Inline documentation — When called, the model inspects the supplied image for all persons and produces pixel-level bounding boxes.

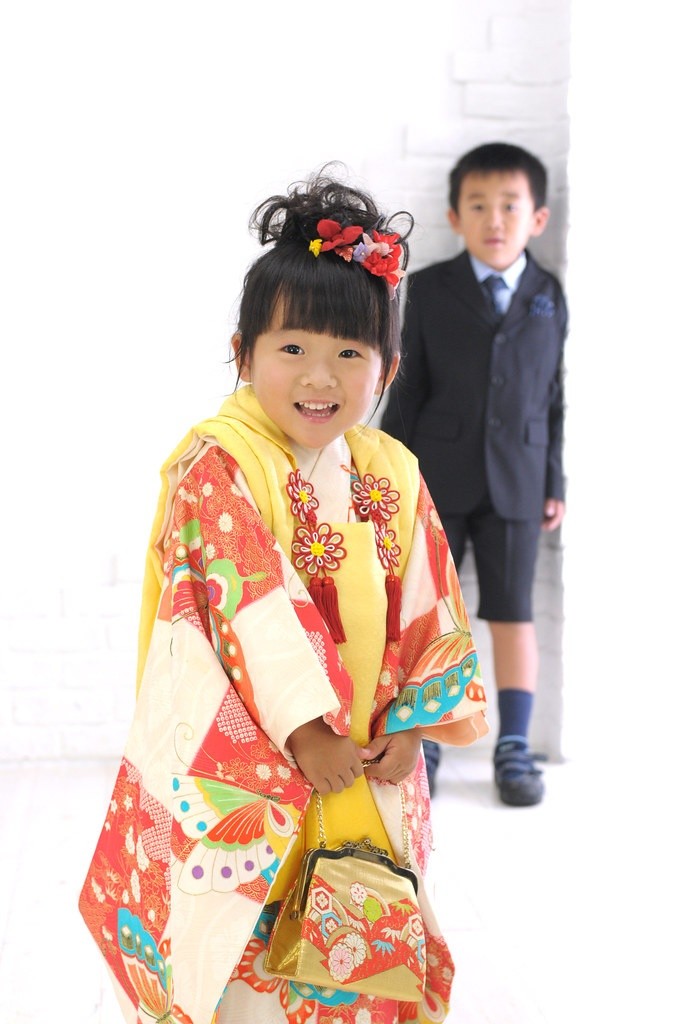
[77,182,487,1024]
[378,140,567,808]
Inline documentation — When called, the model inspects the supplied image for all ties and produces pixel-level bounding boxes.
[483,275,508,319]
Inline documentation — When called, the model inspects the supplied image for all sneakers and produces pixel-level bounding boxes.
[492,741,543,804]
[424,740,441,796]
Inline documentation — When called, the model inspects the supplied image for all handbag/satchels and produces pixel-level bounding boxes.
[264,765,425,1002]
